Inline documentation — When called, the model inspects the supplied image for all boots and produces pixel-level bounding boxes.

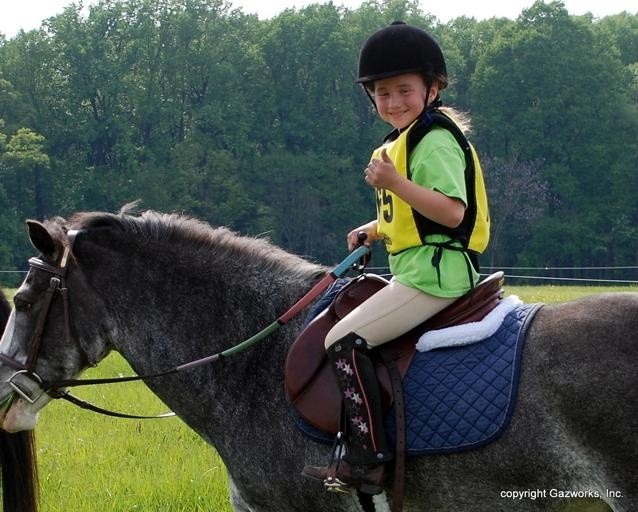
[301,333,390,495]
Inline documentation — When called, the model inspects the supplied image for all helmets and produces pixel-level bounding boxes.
[356,21,448,90]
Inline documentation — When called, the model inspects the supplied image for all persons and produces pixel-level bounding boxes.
[301,21,492,496]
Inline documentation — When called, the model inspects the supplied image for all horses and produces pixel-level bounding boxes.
[0,198,637,511]
